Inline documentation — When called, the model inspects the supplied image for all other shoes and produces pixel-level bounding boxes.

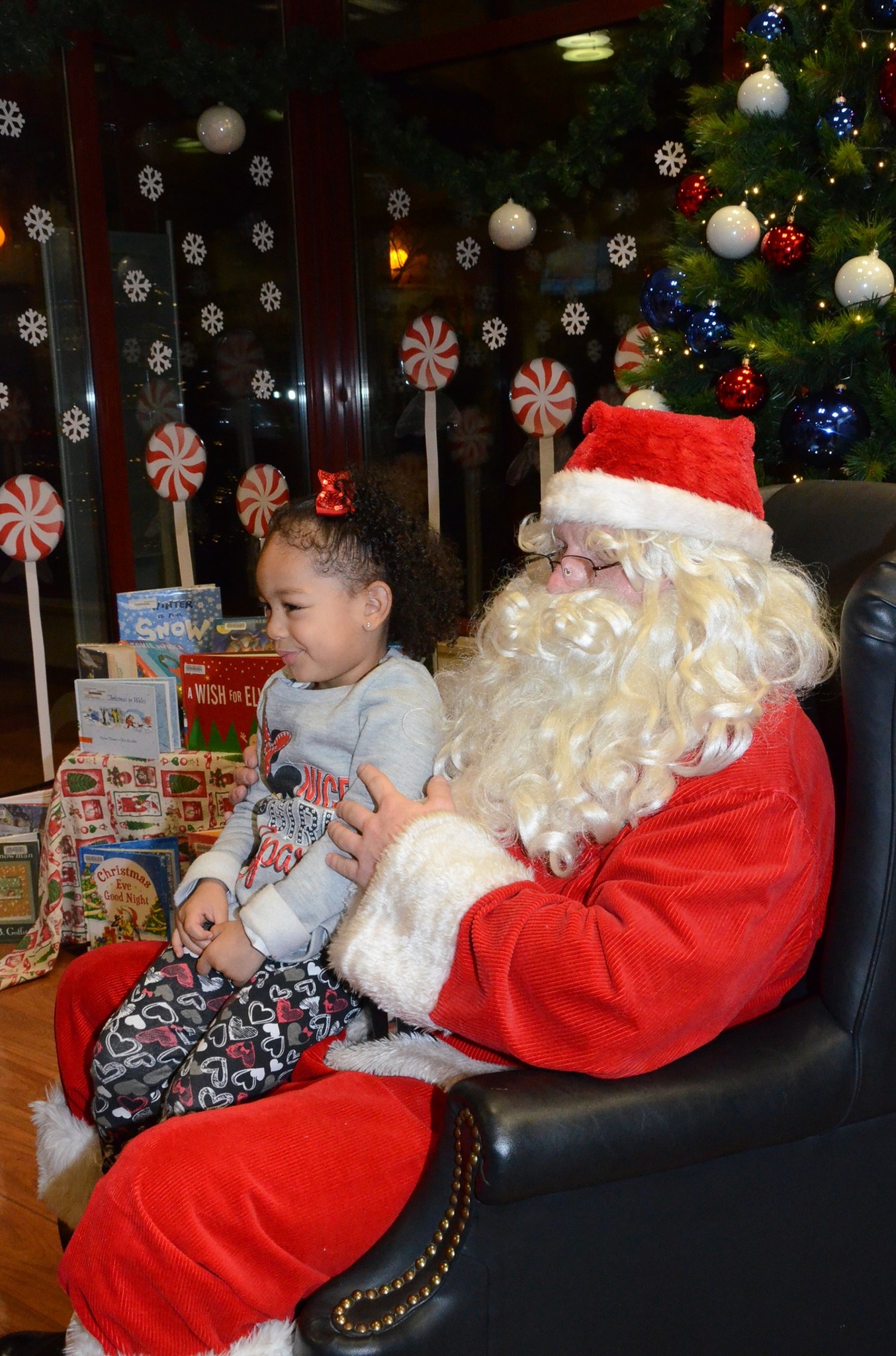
[0,1330,67,1356]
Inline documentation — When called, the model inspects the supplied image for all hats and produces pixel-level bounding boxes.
[538,400,773,563]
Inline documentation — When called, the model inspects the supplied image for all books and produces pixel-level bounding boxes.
[116,582,223,654]
[74,675,181,760]
[211,616,277,654]
[0,831,41,943]
[78,836,181,953]
[187,824,227,866]
[0,788,55,837]
[179,649,288,754]
[76,641,138,679]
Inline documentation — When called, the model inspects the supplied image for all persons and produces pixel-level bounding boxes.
[24,395,844,1356]
[86,465,466,1178]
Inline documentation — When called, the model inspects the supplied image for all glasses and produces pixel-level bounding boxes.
[517,545,621,590]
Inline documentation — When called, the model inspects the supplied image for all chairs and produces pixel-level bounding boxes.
[291,478,896,1353]
